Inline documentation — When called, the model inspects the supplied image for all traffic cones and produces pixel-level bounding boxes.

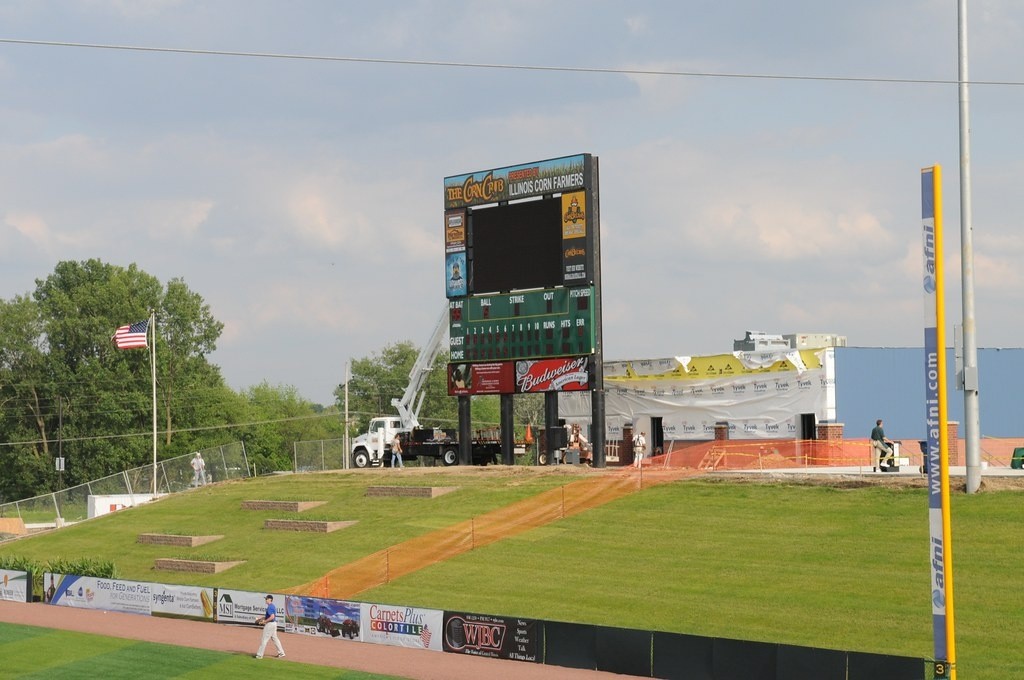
[525,424,533,442]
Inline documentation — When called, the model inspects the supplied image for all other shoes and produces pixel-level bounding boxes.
[400,465,405,468]
[880,462,890,467]
[875,469,882,473]
[255,655,263,659]
[275,654,286,658]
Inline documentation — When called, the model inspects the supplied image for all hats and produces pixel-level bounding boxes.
[264,595,273,599]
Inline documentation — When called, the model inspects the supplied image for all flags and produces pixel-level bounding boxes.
[675,355,691,373]
[787,351,807,375]
[115,318,150,348]
[526,424,531,441]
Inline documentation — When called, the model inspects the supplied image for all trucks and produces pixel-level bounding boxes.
[351,300,533,468]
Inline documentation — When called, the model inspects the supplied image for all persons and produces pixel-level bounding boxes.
[871,420,893,471]
[391,434,405,467]
[632,432,645,467]
[654,446,662,455]
[191,453,205,488]
[47,574,55,601]
[254,595,285,659]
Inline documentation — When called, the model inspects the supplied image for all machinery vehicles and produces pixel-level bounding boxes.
[539,423,619,466]
